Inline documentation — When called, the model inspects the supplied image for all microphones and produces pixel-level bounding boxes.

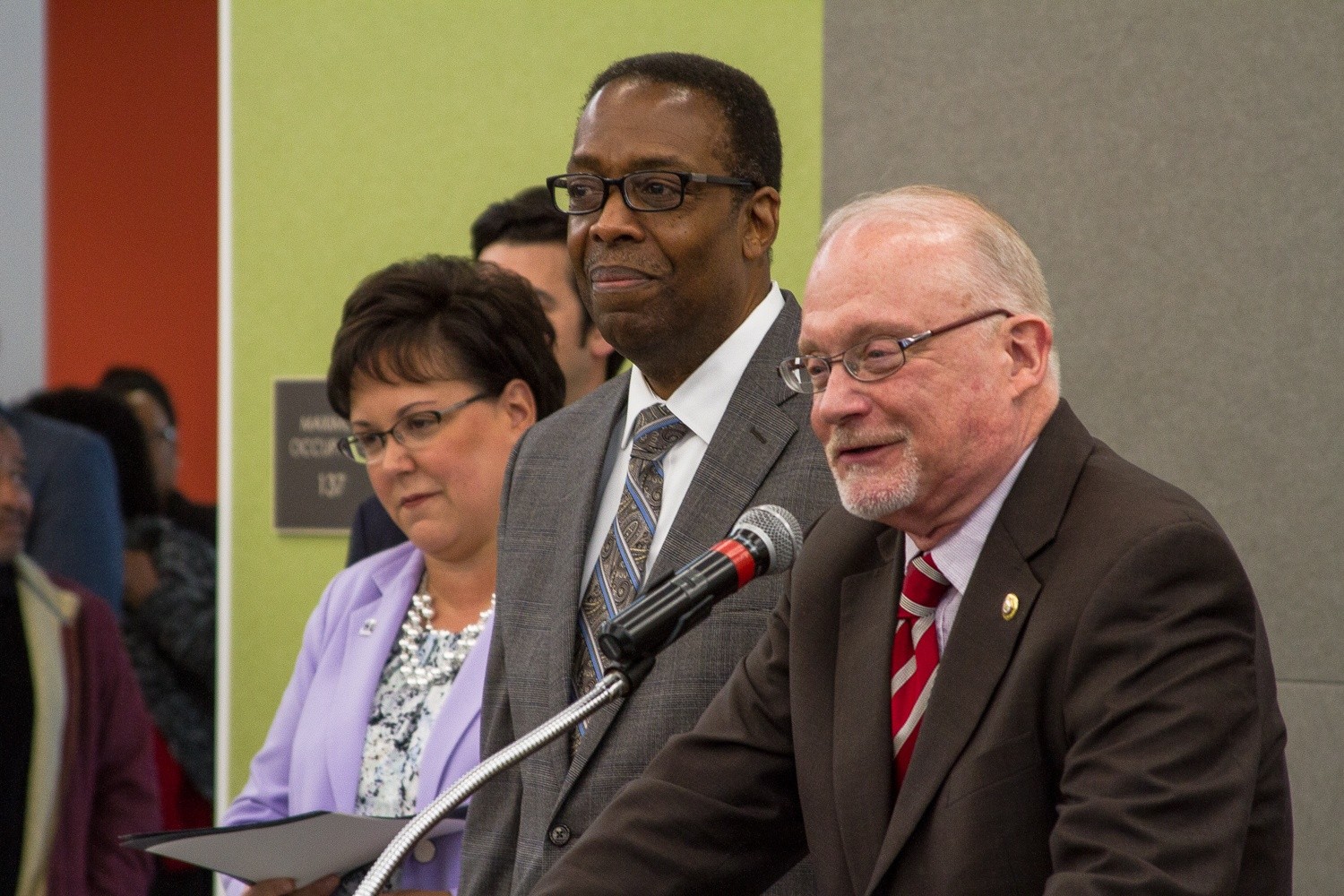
[595,502,804,666]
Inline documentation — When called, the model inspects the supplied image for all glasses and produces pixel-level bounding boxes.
[335,391,503,464]
[546,167,779,213]
[775,308,1015,394]
[145,427,173,447]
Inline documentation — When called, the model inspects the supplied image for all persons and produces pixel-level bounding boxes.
[0,361,215,896]
[525,184,1296,896]
[454,53,847,896]
[345,181,624,573]
[210,254,568,896]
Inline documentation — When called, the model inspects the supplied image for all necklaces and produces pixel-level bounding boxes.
[399,563,499,689]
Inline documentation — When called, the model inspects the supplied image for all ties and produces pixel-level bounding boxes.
[888,551,954,804]
[568,402,690,768]
